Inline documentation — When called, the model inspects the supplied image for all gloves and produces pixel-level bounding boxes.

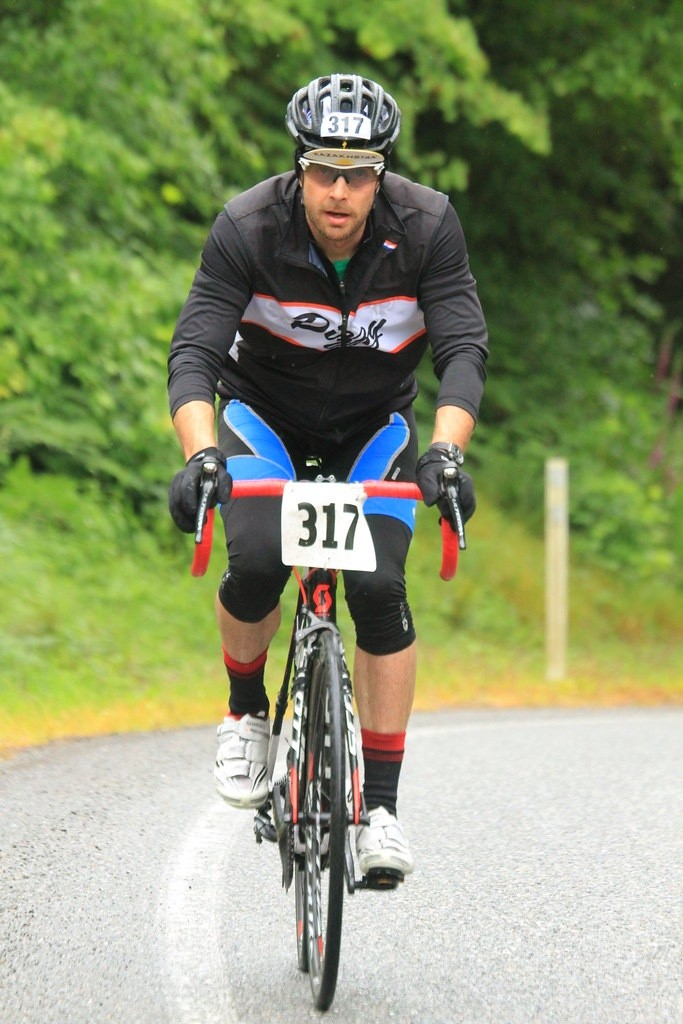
[415,449,476,532]
[169,447,233,533]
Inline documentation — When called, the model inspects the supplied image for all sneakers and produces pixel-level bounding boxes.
[356,805,415,874]
[214,712,269,809]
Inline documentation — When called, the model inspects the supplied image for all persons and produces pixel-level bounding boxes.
[165,74,490,877]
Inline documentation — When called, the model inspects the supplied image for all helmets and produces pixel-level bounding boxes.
[284,73,402,175]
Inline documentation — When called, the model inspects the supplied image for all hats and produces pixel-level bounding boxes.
[301,146,385,168]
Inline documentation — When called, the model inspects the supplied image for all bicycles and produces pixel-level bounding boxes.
[185,444,470,1013]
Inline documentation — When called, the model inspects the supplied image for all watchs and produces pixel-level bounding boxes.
[427,441,466,468]
